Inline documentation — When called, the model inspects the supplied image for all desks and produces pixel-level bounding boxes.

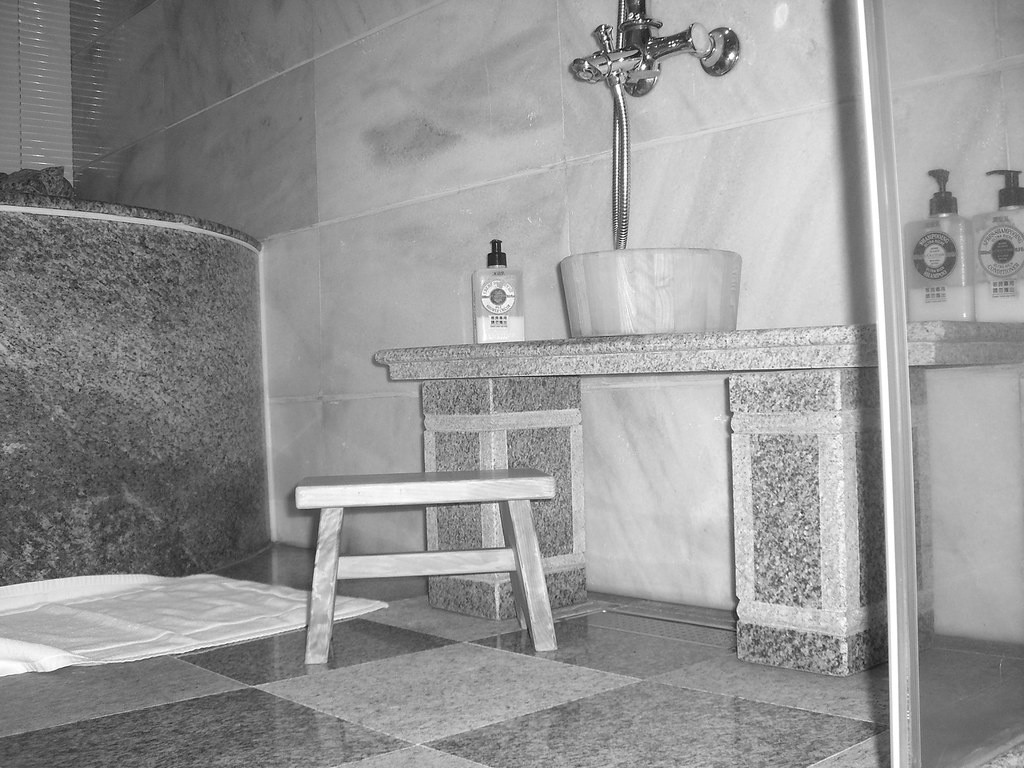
[373,318,1024,678]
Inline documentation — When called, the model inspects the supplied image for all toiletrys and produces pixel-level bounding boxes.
[904,166,972,326]
[469,238,527,344]
[972,168,1024,324]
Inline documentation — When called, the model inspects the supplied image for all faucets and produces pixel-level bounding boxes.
[570,18,662,85]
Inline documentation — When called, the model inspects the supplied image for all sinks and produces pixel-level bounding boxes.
[559,246,740,341]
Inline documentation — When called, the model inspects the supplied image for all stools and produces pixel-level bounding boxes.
[295,470,557,665]
